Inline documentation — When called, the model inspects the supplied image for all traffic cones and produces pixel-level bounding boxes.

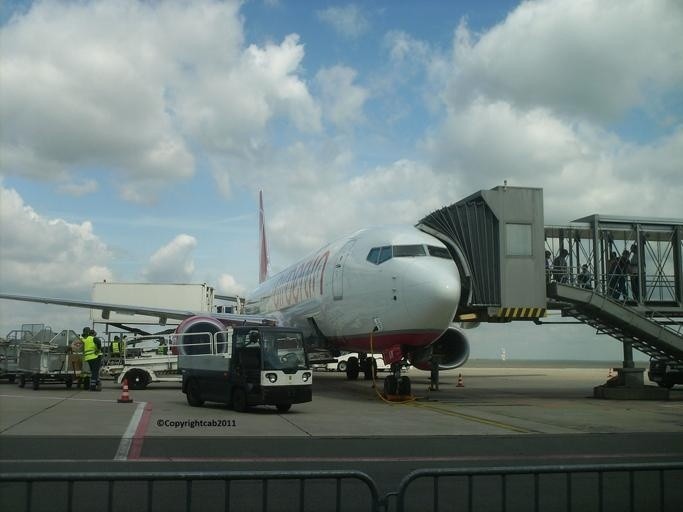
[118,378,133,403]
[456,372,464,387]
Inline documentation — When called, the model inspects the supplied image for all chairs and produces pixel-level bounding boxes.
[240,348,264,381]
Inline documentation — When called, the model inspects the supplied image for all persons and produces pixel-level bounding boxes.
[611,250,632,301]
[577,263,590,287]
[156,337,168,355]
[545,250,551,281]
[608,251,619,296]
[110,335,119,356]
[552,248,570,285]
[78,326,104,392]
[120,334,128,356]
[628,244,639,301]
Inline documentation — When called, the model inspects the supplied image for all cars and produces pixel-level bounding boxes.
[327,352,413,373]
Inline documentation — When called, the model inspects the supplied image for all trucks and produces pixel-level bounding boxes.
[177,313,313,413]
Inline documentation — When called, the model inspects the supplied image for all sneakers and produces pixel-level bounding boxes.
[90,384,101,391]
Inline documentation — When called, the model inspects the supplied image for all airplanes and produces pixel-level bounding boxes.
[0,189,470,405]
